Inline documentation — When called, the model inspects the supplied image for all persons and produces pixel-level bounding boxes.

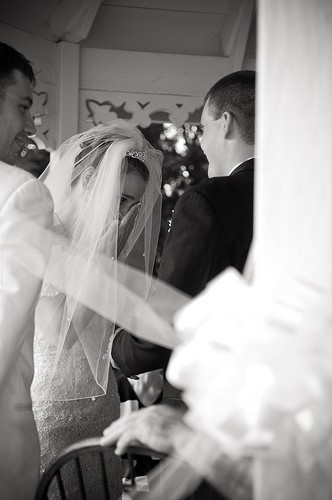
[97,402,332,500]
[27,130,149,500]
[106,68,255,407]
[0,40,54,500]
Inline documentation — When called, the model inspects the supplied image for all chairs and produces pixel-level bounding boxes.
[33,435,160,500]
